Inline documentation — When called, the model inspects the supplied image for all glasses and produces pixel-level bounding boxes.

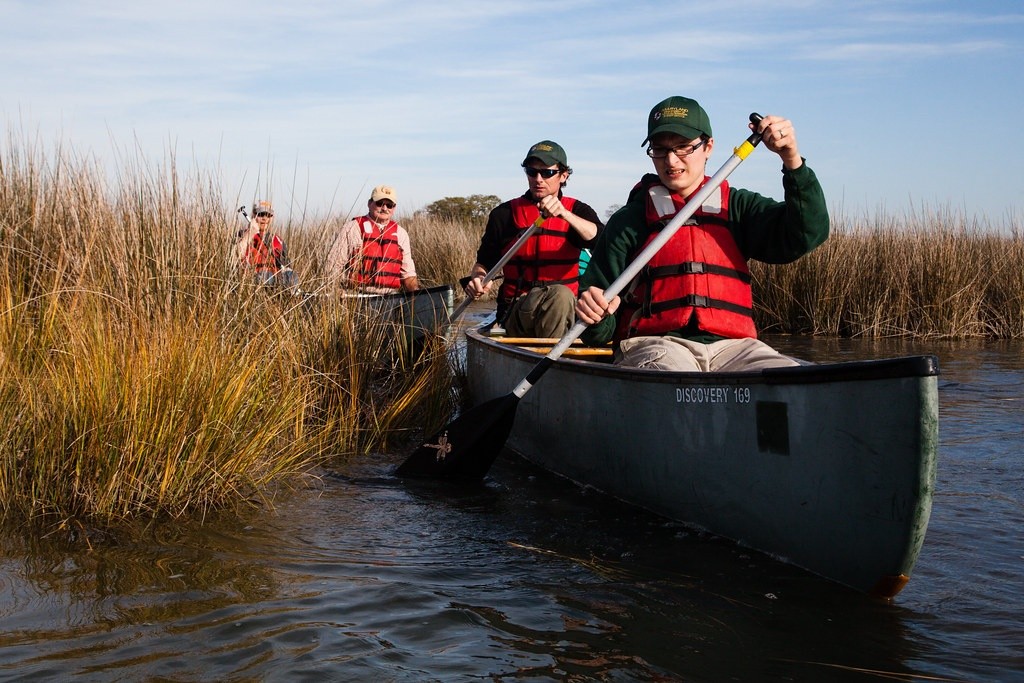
[254,212,273,217]
[526,167,562,178]
[371,198,396,209]
[647,137,709,159]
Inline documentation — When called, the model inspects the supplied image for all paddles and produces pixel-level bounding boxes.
[394,113,782,482]
[433,208,551,335]
[237,205,299,292]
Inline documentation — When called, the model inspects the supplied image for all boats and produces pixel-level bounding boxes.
[465,309,941,606]
[303,284,455,362]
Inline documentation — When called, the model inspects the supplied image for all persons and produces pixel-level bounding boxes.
[326,186,417,294]
[465,140,605,338]
[576,96,829,372]
[232,201,302,291]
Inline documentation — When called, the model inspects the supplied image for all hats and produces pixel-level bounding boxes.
[371,185,396,205]
[641,96,712,148]
[523,140,567,168]
[253,200,273,215]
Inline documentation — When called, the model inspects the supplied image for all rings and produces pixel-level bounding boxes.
[779,130,783,139]
